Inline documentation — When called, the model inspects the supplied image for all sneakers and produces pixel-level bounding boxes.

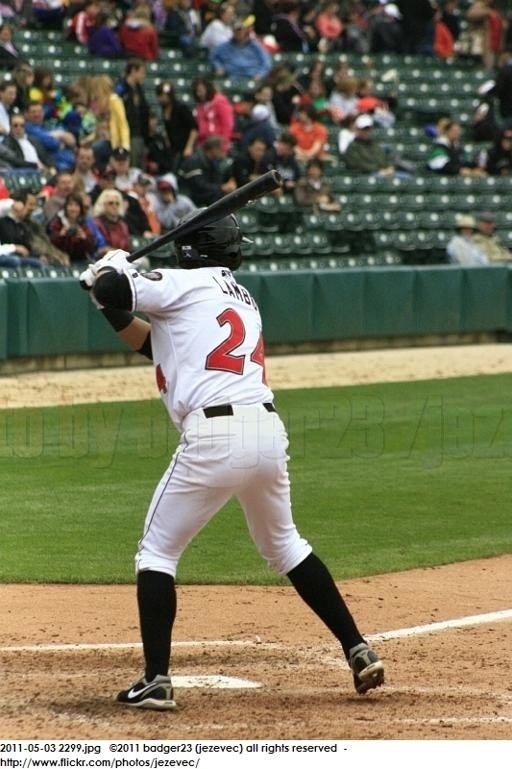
[348,642,385,693]
[118,675,177,710]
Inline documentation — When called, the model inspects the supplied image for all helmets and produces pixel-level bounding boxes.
[174,206,242,270]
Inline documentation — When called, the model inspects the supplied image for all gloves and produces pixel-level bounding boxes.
[88,248,139,311]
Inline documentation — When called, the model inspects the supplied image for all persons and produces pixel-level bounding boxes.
[78,204,386,710]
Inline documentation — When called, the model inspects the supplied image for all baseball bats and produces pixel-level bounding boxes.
[81,170,282,290]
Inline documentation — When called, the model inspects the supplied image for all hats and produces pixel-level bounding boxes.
[354,114,372,128]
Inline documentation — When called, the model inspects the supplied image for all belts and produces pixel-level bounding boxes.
[202,403,276,418]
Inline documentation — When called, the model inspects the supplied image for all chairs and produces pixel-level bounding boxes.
[0,22,512,280]
